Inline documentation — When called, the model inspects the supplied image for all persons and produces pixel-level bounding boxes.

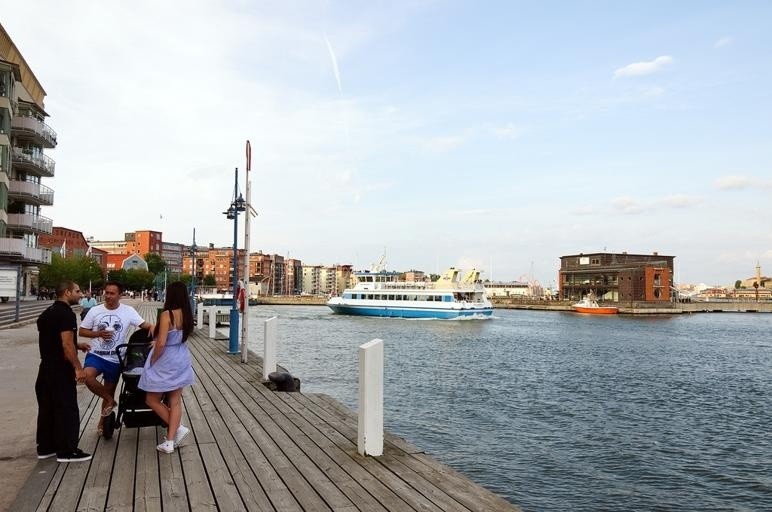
[34,278,93,464]
[80,291,96,320]
[37,285,166,304]
[78,281,156,435]
[136,280,196,455]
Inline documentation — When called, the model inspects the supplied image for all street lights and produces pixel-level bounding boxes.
[223,168,246,354]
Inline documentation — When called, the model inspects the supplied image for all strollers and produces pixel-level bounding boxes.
[103,328,170,438]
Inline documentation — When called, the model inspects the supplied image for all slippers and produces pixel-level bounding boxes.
[100,407,113,416]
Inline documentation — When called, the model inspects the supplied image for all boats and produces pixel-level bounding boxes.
[325,266,493,321]
[572,298,618,314]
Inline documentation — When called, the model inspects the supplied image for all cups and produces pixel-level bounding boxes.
[104,327,114,340]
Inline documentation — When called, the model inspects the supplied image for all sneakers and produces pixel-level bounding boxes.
[174,425,190,449]
[56,448,92,463]
[36,445,56,459]
[157,440,174,454]
